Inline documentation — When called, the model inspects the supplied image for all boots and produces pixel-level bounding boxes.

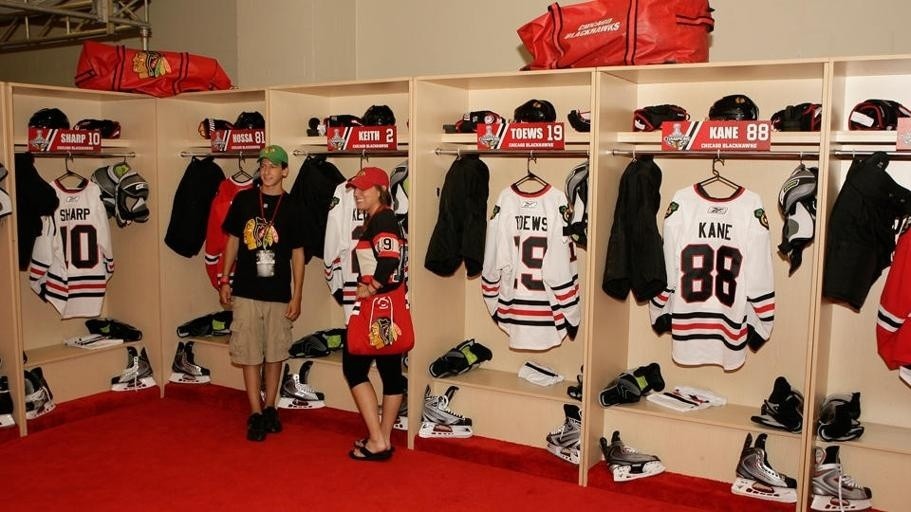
[422,386,471,425]
[279,362,325,400]
[600,430,660,468]
[811,447,872,499]
[173,340,210,376]
[547,404,582,449]
[22,368,53,411]
[0,375,14,416]
[736,434,796,488]
[112,347,153,384]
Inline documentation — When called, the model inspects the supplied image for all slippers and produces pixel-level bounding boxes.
[349,439,393,460]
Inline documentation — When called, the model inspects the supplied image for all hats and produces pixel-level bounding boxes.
[345,167,388,191]
[257,145,288,166]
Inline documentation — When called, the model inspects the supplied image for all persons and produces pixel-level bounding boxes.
[334,167,409,460]
[218,145,306,442]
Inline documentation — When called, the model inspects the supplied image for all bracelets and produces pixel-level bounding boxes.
[219,277,231,285]
[369,282,379,291]
[222,275,229,276]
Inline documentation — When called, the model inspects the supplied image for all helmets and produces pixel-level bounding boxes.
[363,105,396,127]
[515,99,556,122]
[234,112,264,131]
[30,107,69,130]
[709,94,758,121]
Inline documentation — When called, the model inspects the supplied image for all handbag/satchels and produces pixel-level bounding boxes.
[76,41,230,97]
[518,0,714,69]
[348,288,415,355]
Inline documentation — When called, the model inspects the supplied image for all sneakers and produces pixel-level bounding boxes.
[262,406,281,433]
[247,412,265,440]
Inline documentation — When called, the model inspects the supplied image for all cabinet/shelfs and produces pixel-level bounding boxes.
[407,67,596,488]
[802,54,911,512]
[585,58,829,512]
[264,75,413,428]
[0,81,25,436]
[158,84,268,393]
[6,81,161,436]
